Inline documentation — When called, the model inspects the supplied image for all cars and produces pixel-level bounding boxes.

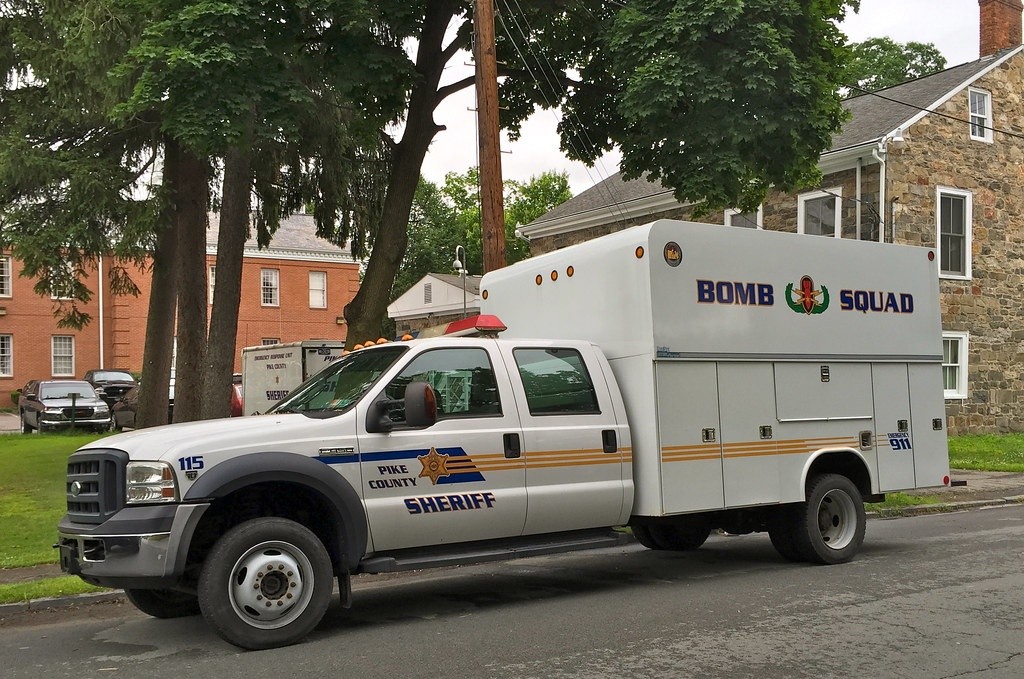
[17,379,110,435]
[110,369,175,433]
[230,372,243,418]
[82,369,139,409]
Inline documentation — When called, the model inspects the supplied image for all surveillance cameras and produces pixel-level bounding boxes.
[453,260,462,270]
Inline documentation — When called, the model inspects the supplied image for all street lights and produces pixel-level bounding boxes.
[454,245,468,318]
[874,127,906,243]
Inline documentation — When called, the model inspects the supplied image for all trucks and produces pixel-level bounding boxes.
[241,340,346,422]
[52,218,970,651]
